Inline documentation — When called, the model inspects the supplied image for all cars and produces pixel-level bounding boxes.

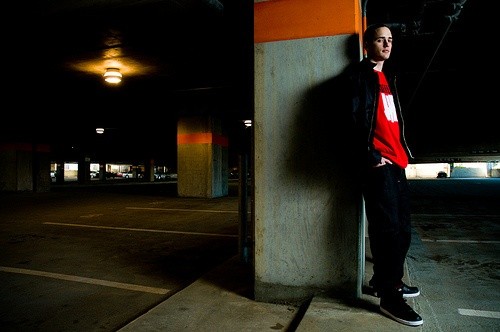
[89,169,178,181]
[49,169,58,179]
[437,171,447,179]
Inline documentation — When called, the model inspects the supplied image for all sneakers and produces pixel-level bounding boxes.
[374,283,420,297]
[380,295,423,325]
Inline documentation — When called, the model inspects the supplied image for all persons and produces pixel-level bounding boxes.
[339,24,423,326]
